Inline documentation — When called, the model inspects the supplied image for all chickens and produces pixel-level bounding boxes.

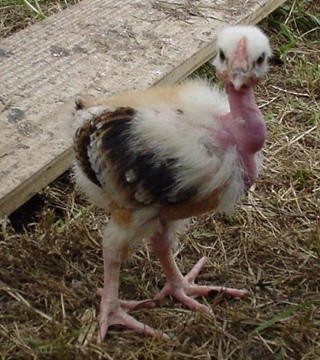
[70,24,272,347]
